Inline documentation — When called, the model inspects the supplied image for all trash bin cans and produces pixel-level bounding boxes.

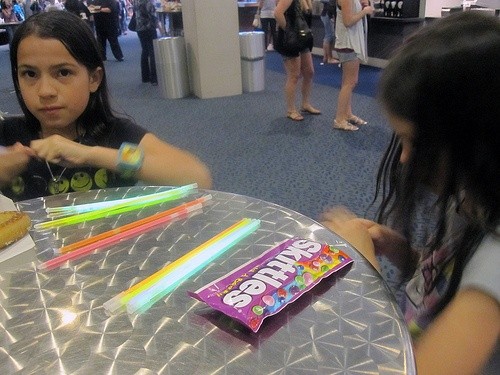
[240,30,265,90]
[154,36,189,100]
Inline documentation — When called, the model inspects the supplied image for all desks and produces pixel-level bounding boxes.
[156,9,182,36]
[0,186,416,375]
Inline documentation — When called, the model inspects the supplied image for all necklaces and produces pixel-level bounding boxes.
[39,124,86,195]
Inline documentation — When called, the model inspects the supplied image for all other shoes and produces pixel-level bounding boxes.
[119,57,124,61]
[151,78,157,82]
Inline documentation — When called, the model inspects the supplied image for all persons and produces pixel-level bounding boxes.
[0,0,168,86]
[0,10,214,203]
[258,0,377,131]
[323,10,500,375]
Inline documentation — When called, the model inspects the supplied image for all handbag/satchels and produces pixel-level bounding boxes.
[128,14,136,31]
[253,13,263,30]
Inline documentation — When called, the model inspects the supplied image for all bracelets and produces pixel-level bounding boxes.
[115,141,145,180]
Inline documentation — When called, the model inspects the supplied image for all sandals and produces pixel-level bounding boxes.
[301,105,320,114]
[288,111,304,121]
[334,119,359,130]
[350,115,367,125]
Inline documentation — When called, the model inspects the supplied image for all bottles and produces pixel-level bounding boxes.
[385,1,404,18]
